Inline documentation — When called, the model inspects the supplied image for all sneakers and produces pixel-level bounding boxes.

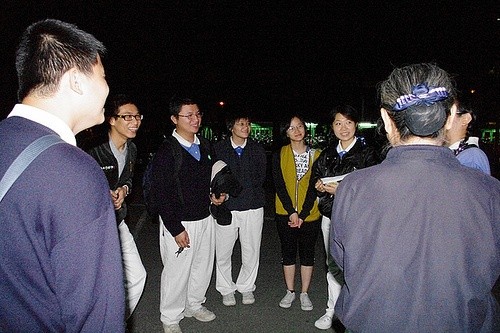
[242,291,255,304]
[300,292,313,310]
[185,306,216,321]
[163,323,182,333]
[222,291,236,305]
[315,313,332,329]
[279,290,296,308]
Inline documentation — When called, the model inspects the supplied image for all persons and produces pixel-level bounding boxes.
[149,94,230,333]
[444,90,491,174]
[215,110,268,306]
[273,112,325,311]
[329,64,500,333]
[311,104,382,330]
[81,90,148,324]
[0,18,129,333]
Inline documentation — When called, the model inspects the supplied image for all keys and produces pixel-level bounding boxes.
[176,247,183,258]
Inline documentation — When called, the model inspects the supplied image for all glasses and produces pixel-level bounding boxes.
[288,123,304,130]
[178,111,203,119]
[116,114,143,120]
